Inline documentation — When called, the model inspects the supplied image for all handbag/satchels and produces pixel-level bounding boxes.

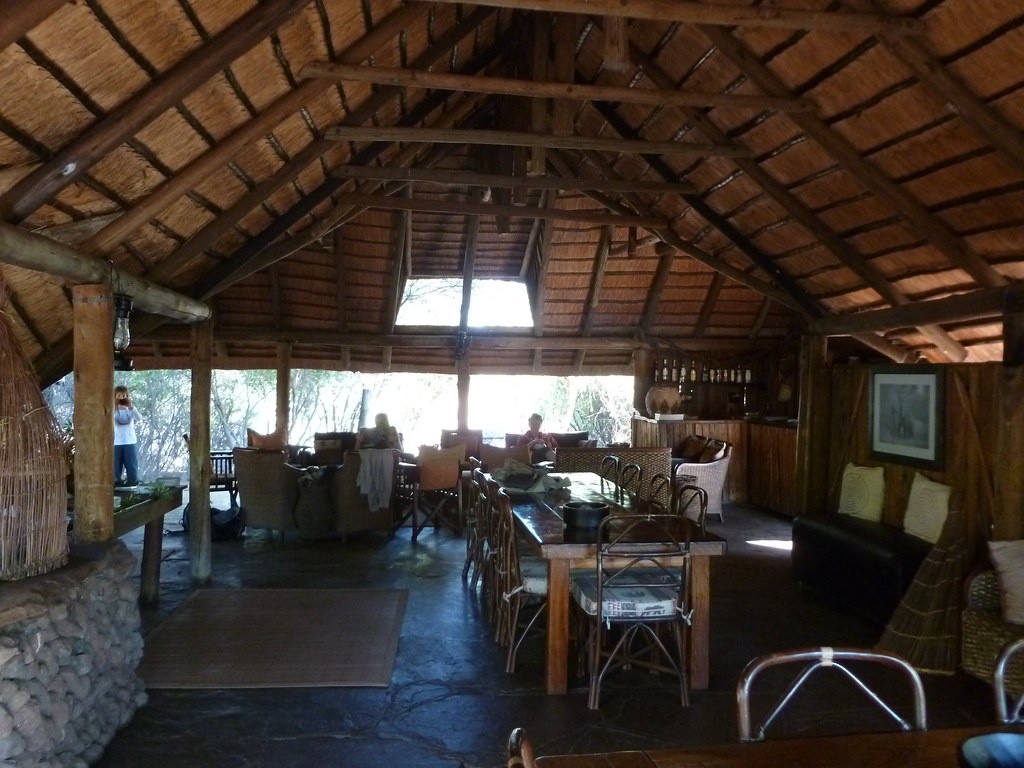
[163,502,246,541]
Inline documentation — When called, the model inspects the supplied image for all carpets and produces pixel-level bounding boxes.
[135,586,409,690]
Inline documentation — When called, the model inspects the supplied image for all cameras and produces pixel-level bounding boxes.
[118,399,124,405]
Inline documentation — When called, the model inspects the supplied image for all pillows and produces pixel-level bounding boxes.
[986,539,1024,626]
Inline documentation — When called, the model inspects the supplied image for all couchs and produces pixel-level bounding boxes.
[672,435,712,475]
[673,438,733,530]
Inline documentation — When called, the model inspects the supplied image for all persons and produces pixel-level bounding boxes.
[113,385,141,489]
[516,414,559,467]
[353,413,403,451]
[516,434,555,467]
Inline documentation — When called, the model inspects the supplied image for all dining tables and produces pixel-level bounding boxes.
[480,472,728,696]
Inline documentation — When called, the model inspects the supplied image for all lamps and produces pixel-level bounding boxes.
[113,294,136,372]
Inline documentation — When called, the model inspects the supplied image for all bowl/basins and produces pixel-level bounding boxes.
[563,500,610,528]
[156,477,181,487]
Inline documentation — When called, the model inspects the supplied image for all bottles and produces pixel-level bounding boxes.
[654,359,659,382]
[724,370,727,382]
[680,363,686,382]
[672,360,678,382]
[736,362,742,383]
[690,361,696,384]
[662,358,669,383]
[702,364,708,382]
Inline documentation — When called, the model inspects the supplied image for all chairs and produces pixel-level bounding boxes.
[600,456,622,485]
[462,456,483,591]
[736,647,928,744]
[993,638,1024,725]
[233,446,305,542]
[620,485,709,676]
[647,472,672,514]
[335,448,399,543]
[183,433,239,510]
[619,463,643,497]
[500,487,592,677]
[470,468,490,601]
[412,457,468,544]
[569,512,692,710]
[485,477,537,643]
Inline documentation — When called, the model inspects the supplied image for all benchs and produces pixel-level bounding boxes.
[555,447,673,513]
[793,503,933,629]
[959,558,1024,705]
[505,431,599,467]
[313,426,403,464]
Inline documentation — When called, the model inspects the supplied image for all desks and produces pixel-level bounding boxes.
[730,412,799,519]
[68,484,189,608]
[631,413,750,508]
[536,724,1024,767]
[291,463,418,517]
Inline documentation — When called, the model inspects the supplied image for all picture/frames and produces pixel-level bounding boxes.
[865,362,948,474]
